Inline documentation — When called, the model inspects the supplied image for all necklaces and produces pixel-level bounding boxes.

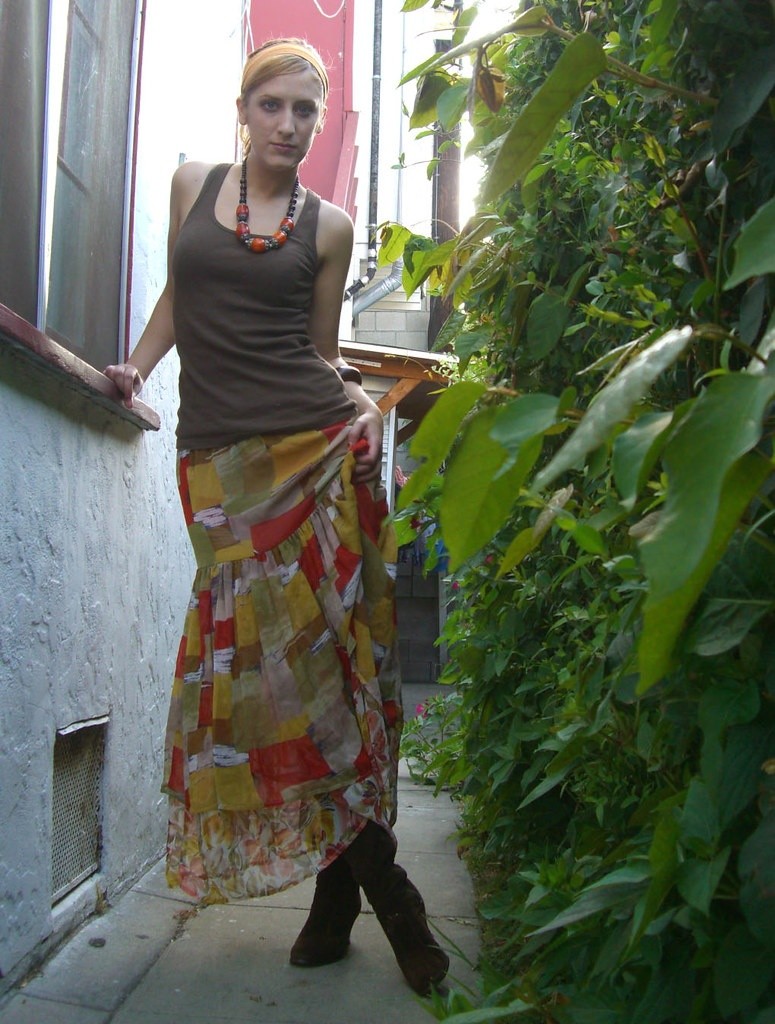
[236,157,300,252]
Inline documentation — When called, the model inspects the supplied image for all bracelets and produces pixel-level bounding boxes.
[336,366,362,385]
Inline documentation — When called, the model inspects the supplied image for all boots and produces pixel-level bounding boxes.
[344,819,449,995]
[290,853,361,967]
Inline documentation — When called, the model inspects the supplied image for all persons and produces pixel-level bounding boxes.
[104,37,450,993]
